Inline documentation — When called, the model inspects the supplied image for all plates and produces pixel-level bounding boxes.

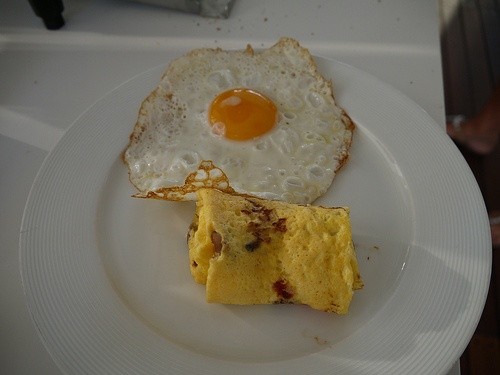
[18,50,494,375]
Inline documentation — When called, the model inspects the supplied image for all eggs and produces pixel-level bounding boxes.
[123,38,354,205]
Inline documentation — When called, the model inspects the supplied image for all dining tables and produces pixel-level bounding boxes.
[0,1,467,375]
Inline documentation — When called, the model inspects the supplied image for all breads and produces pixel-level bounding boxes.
[184,187,363,314]
